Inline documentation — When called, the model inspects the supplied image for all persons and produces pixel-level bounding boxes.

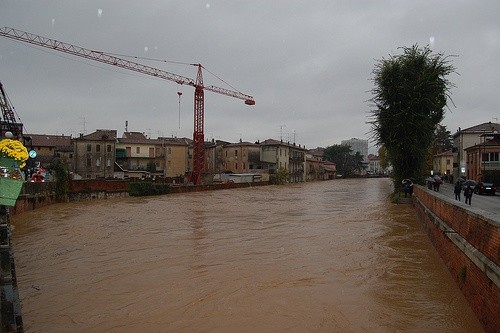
[454,180,462,201]
[464,185,474,206]
[427,174,453,192]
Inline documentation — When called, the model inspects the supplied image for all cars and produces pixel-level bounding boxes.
[474,183,496,195]
[460,179,478,190]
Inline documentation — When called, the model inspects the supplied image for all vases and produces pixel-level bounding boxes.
[0,155,19,169]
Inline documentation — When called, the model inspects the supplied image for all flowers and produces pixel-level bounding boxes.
[0,139,29,168]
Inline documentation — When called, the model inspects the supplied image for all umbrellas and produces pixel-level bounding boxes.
[425,177,436,182]
[464,179,478,186]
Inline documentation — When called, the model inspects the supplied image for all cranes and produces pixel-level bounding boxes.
[0,83,34,182]
[1,22,258,184]
